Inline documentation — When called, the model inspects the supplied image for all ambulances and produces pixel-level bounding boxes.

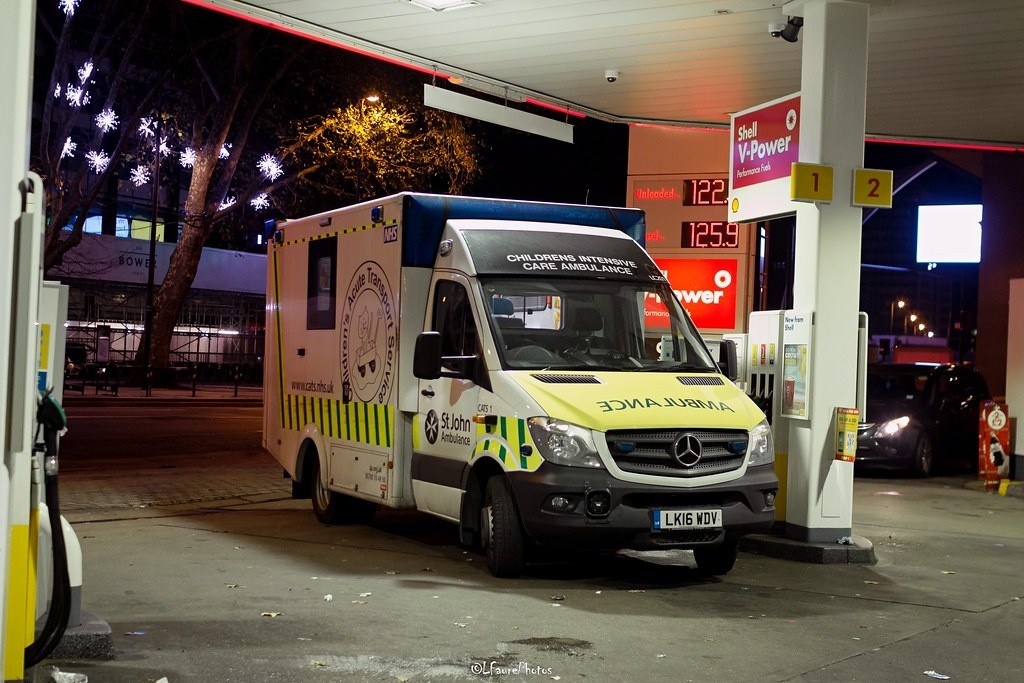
[256,190,781,576]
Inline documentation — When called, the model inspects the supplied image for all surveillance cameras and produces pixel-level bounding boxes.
[605,70,618,82]
[768,23,785,38]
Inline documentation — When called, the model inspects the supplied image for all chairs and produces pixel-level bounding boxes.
[490,297,523,328]
[569,308,616,352]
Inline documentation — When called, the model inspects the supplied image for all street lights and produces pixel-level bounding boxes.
[890,297,935,338]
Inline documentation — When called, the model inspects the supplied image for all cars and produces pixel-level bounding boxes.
[855,359,992,479]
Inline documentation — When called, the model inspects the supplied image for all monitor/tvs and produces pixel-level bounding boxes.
[917,204,983,262]
